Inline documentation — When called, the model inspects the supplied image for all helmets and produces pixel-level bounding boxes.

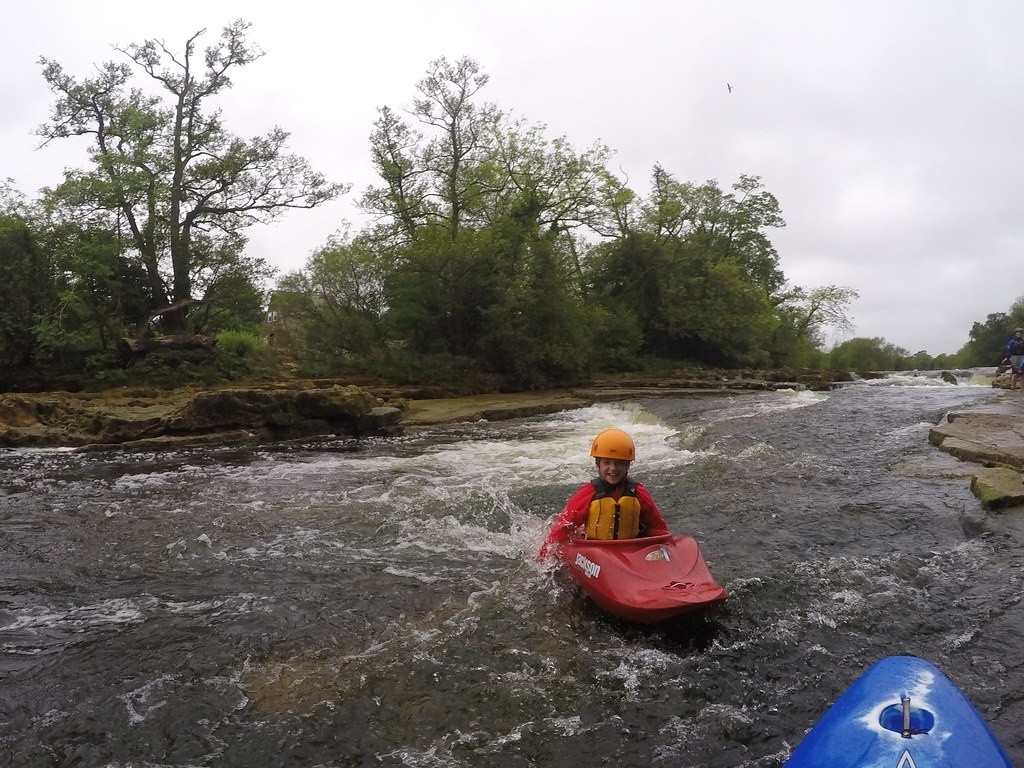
[589,430,635,460]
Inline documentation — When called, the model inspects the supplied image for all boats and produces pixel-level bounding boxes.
[548,511,729,629]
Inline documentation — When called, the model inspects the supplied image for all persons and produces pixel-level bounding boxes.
[536,429,668,564]
[1002,328,1024,390]
